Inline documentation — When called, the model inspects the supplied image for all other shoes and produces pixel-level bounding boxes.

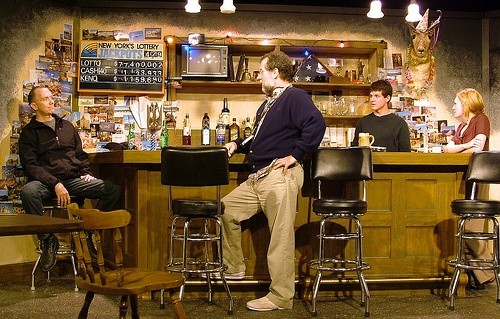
[466,280,485,291]
[246,295,285,311]
[201,267,245,279]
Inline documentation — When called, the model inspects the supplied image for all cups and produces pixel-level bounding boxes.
[432,147,442,153]
[358,133,375,145]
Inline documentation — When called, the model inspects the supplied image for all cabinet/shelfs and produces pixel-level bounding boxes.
[165,33,388,150]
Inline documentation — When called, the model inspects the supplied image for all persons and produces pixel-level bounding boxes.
[441,87,496,284]
[18,86,120,273]
[202,49,327,311]
[350,80,412,152]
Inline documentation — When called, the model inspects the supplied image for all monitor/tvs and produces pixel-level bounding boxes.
[182,45,228,77]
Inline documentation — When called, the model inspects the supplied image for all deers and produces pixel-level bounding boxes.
[405,7,442,99]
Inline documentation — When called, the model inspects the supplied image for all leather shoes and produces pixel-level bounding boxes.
[39,235,59,271]
[86,231,98,257]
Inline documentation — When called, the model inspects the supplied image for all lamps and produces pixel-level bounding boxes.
[405,0,423,22]
[367,0,384,18]
[220,0,236,14]
[185,0,201,13]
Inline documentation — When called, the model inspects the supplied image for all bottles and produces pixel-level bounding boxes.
[243,69,251,82]
[316,95,371,116]
[252,117,256,130]
[230,118,239,141]
[160,120,169,150]
[336,68,372,84]
[215,115,226,146]
[245,118,251,139]
[201,112,210,145]
[127,122,135,149]
[241,121,246,140]
[182,113,191,145]
[222,97,230,129]
[253,71,260,81]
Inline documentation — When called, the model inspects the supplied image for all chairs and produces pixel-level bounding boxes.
[159,146,233,315]
[29,193,85,292]
[66,202,186,319]
[304,145,373,317]
[448,150,500,310]
